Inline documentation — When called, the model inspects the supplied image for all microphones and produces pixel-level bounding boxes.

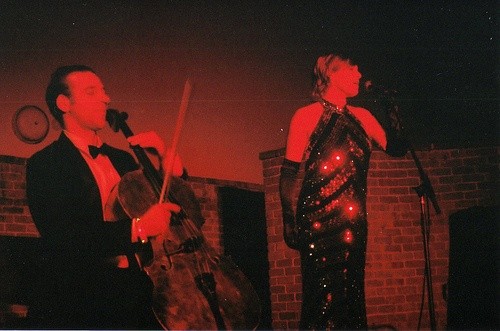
[366,81,397,96]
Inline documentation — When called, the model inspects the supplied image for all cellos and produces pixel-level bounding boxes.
[104,108,263,331]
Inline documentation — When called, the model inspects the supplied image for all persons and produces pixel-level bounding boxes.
[279,50,405,331]
[24,64,189,331]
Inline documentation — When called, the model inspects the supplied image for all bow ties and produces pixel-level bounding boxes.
[88,142,113,159]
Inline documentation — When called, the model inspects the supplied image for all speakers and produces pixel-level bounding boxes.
[445,207,500,331]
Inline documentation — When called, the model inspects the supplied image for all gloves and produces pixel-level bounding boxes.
[381,89,405,157]
[279,158,300,250]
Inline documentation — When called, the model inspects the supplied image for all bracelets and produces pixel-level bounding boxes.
[136,218,148,243]
